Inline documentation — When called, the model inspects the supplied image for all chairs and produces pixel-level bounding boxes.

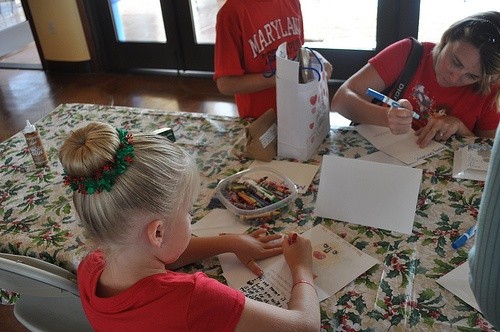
[0,253,93,332]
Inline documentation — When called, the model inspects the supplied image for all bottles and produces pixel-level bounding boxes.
[22,119,48,167]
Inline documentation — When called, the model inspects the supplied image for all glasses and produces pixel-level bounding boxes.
[464,18,500,47]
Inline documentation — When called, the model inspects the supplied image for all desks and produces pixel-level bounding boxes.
[0,103,500,332]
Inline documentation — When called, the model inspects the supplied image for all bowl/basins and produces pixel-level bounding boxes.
[215,166,300,225]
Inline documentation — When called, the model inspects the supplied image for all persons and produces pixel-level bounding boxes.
[214,0,333,121]
[59,122,320,332]
[330,11,500,147]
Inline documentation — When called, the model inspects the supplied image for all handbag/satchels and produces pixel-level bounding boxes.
[274,41,332,162]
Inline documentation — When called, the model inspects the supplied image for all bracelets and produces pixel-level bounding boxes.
[292,280,318,294]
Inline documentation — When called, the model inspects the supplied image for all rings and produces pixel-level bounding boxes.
[438,131,445,137]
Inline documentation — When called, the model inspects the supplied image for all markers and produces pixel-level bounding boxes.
[451,222,477,250]
[365,87,422,121]
